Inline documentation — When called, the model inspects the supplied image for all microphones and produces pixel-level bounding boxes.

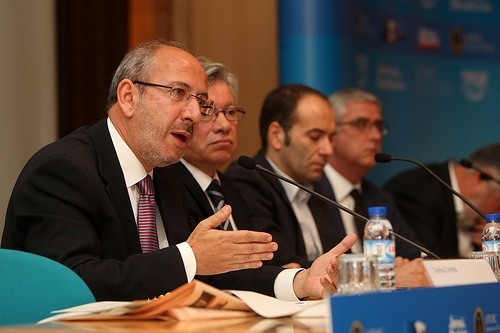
[238,155,443,261]
[375,153,488,221]
[459,158,500,186]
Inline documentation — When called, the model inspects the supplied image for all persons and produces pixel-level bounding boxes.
[0,39,358,301]
[222,84,500,287]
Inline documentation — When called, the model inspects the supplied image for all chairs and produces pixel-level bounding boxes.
[0,246,96,325]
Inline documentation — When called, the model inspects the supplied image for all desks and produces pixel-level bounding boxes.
[0,313,332,333]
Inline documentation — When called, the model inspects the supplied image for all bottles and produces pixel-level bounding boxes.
[362,207,395,291]
[484,214,500,274]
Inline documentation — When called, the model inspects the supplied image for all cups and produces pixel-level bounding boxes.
[336,255,380,295]
[470,251,500,279]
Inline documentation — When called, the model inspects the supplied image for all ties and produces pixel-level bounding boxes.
[206,179,235,231]
[137,175,160,254]
[350,189,370,254]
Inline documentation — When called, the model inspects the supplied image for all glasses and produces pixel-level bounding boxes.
[198,108,245,122]
[336,117,383,132]
[133,80,215,116]
